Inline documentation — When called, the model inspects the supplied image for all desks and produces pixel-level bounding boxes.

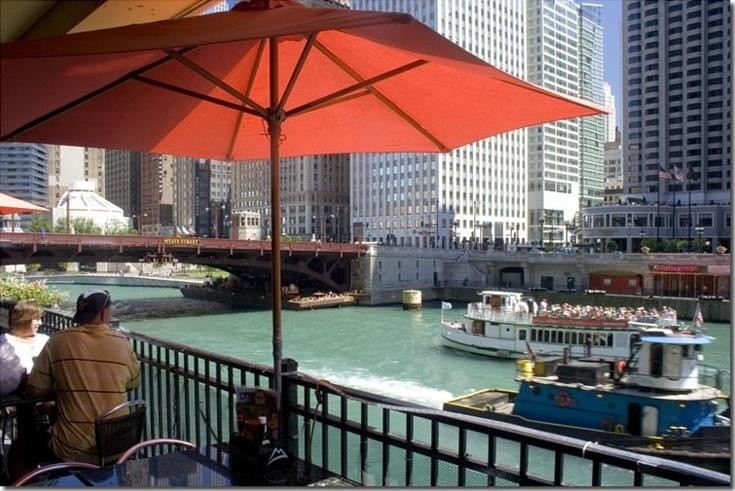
[21,439,357,486]
[1,392,56,479]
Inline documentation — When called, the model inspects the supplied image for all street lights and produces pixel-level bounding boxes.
[205,205,227,239]
[563,220,570,246]
[312,215,335,243]
[131,214,147,236]
[640,227,647,248]
[538,217,544,250]
[695,224,705,252]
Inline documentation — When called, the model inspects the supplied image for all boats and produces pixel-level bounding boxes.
[179,277,272,310]
[282,284,356,313]
[442,319,733,477]
[436,290,701,363]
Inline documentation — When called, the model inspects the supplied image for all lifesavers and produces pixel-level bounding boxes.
[618,361,627,375]
[556,391,571,407]
[616,425,625,434]
[598,419,614,432]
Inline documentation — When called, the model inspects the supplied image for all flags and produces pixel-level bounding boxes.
[673,165,684,183]
[689,166,703,180]
[693,306,704,331]
[657,165,672,180]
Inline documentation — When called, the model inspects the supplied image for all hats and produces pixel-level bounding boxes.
[70,287,112,324]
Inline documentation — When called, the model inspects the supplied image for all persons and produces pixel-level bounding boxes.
[532,298,676,327]
[0,299,57,414]
[6,289,141,478]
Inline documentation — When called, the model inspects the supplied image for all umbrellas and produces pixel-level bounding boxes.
[0,195,49,221]
[0,0,609,446]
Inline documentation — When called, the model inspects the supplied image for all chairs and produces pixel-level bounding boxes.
[95,399,149,470]
[2,408,17,453]
[13,461,101,487]
[116,437,198,464]
